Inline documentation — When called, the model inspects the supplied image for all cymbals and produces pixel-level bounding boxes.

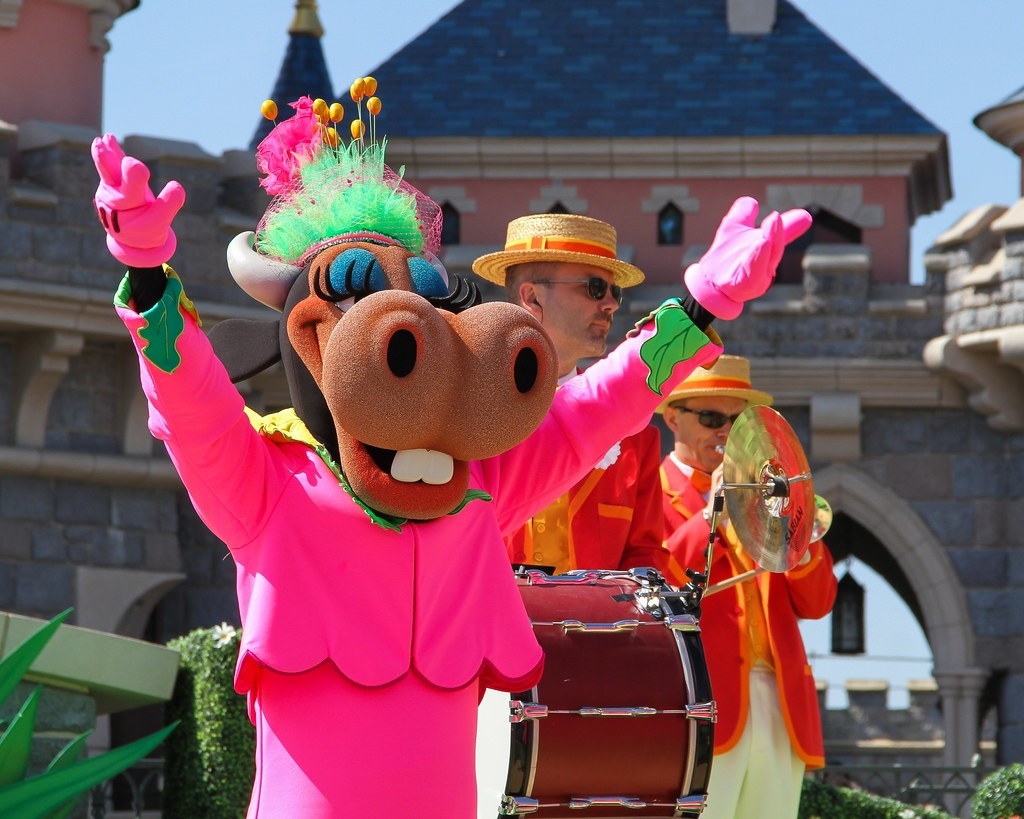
[723,405,815,573]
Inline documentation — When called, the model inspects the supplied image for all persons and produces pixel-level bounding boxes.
[656,356,838,819]
[473,214,669,574]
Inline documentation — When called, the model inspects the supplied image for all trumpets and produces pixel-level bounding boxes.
[715,444,833,544]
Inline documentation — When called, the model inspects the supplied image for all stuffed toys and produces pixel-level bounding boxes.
[92,76,812,819]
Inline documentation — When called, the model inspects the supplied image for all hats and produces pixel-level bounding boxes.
[654,353,773,416]
[472,214,644,287]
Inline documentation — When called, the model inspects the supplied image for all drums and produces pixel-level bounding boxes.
[475,564,718,819]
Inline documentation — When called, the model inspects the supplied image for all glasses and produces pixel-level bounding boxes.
[528,278,622,303]
[673,404,740,429]
